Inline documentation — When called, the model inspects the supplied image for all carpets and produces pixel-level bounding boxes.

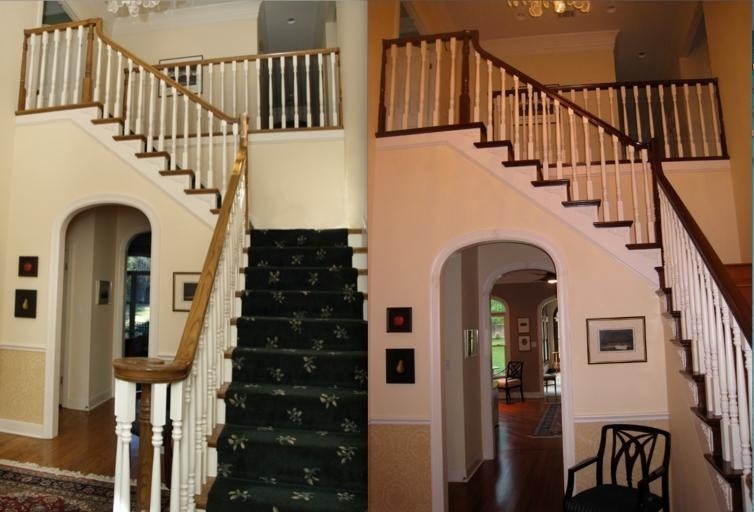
[0,458,171,512]
[532,401,562,439]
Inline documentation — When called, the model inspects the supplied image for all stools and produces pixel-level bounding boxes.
[543,373,556,396]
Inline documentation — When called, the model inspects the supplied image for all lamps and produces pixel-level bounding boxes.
[508,0,591,18]
[105,0,158,15]
[546,271,557,284]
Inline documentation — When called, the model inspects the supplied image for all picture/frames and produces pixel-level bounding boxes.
[517,334,531,352]
[511,82,561,127]
[585,316,649,365]
[158,53,203,99]
[171,271,201,312]
[516,317,531,334]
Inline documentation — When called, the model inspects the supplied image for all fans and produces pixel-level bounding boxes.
[529,273,556,283]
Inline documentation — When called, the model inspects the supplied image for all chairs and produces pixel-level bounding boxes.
[562,423,671,512]
[495,360,525,404]
